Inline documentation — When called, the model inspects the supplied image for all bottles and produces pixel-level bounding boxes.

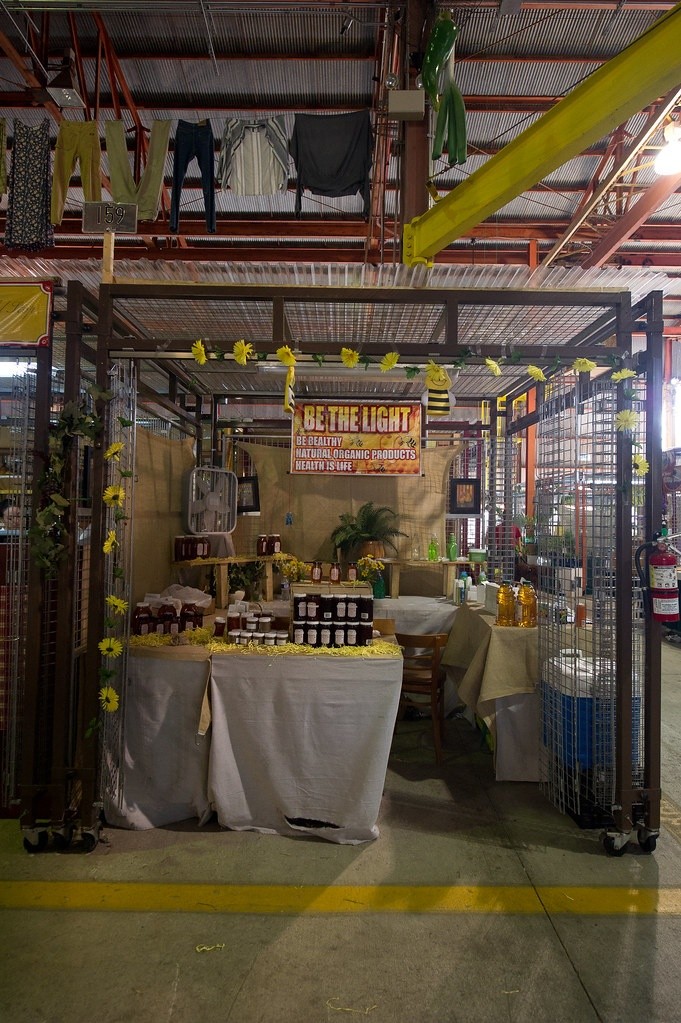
[496,580,516,626]
[411,533,421,560]
[257,533,282,556]
[179,600,198,634]
[156,601,179,635]
[280,576,290,601]
[330,562,340,584]
[445,533,457,561]
[213,610,289,646]
[347,562,358,582]
[311,561,322,584]
[292,593,373,648]
[133,601,153,636]
[174,534,211,561]
[516,581,537,628]
[428,533,439,561]
[373,571,385,599]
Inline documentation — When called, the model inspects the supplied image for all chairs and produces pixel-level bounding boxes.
[395,633,447,765]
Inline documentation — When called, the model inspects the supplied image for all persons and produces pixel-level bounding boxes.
[494,509,523,555]
[0,498,24,532]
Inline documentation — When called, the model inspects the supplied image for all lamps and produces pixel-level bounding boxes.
[45,49,86,109]
[340,17,353,35]
[386,74,426,120]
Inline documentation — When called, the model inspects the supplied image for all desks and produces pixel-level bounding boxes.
[105,596,456,846]
[440,602,583,783]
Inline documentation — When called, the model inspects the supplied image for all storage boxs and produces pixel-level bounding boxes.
[543,649,646,830]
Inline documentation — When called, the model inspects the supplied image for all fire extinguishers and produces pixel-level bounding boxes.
[636,532,681,624]
